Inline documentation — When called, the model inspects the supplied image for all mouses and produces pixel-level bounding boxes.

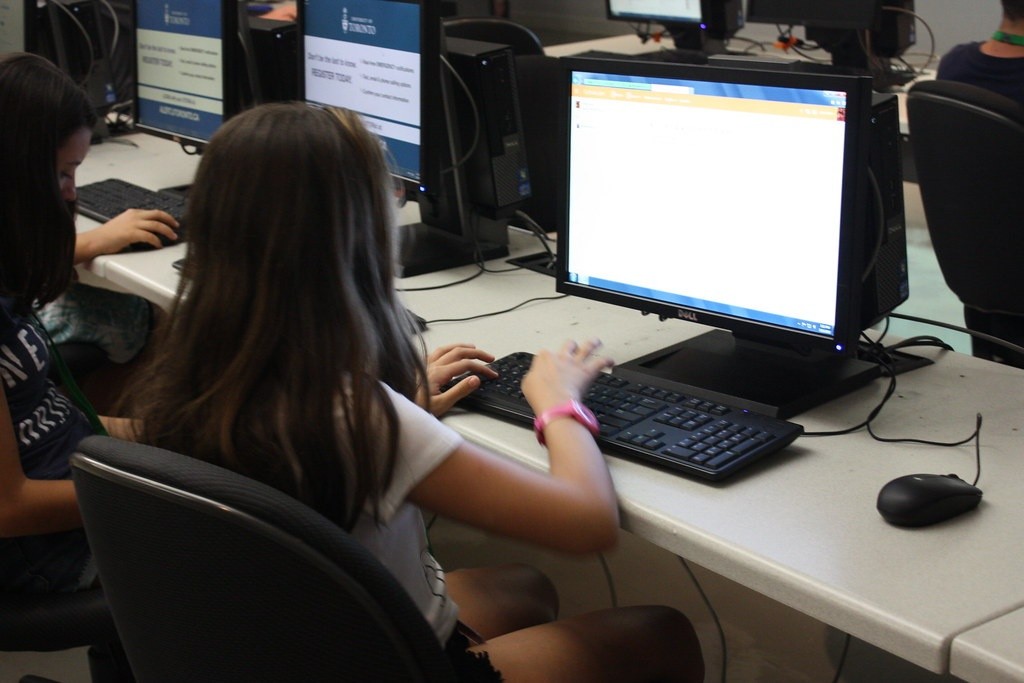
[129,225,183,250]
[876,474,984,526]
[404,307,426,334]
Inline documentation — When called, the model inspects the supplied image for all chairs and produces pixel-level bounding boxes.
[444,19,549,233]
[69,434,454,683]
[908,82,1024,367]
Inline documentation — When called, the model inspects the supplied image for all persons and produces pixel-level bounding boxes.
[36,208,180,420]
[938,0,1024,104]
[127,102,706,683]
[0,55,144,683]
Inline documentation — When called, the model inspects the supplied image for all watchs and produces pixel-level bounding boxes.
[533,400,600,447]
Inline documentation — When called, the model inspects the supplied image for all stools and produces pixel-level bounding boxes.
[0,591,136,683]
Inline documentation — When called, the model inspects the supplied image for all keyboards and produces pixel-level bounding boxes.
[440,352,804,482]
[74,178,199,233]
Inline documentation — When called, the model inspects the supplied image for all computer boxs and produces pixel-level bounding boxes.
[860,92,909,328]
[56,0,118,108]
[444,35,535,220]
[805,0,915,50]
[708,0,744,36]
[248,15,298,105]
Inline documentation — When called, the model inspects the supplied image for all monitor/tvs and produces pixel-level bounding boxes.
[297,0,510,278]
[604,0,756,66]
[132,0,239,151]
[745,0,916,85]
[554,56,881,420]
[0,0,37,56]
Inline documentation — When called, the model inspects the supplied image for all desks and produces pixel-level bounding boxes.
[67,109,1024,683]
[541,29,937,135]
